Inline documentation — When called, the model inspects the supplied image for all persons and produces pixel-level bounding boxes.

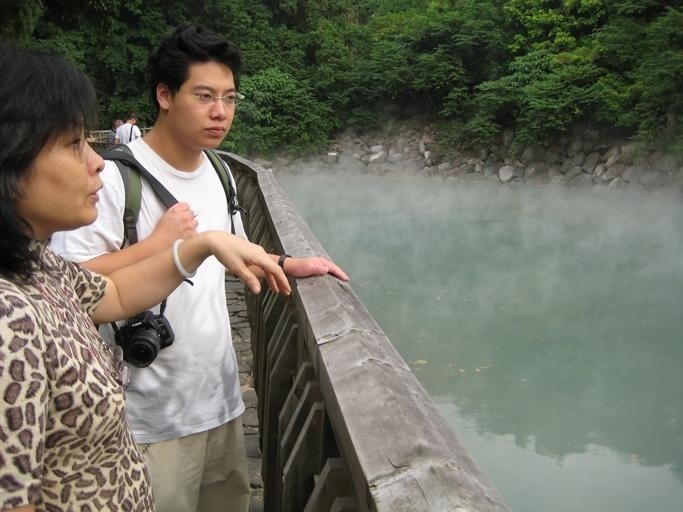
[112,111,142,147]
[104,116,124,144]
[1,49,295,511]
[46,18,349,512]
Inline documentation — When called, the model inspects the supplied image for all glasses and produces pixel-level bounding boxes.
[174,86,247,109]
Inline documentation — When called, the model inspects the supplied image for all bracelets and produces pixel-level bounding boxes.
[172,237,198,278]
[277,252,293,268]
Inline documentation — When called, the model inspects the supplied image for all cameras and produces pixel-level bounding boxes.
[113,310,175,369]
